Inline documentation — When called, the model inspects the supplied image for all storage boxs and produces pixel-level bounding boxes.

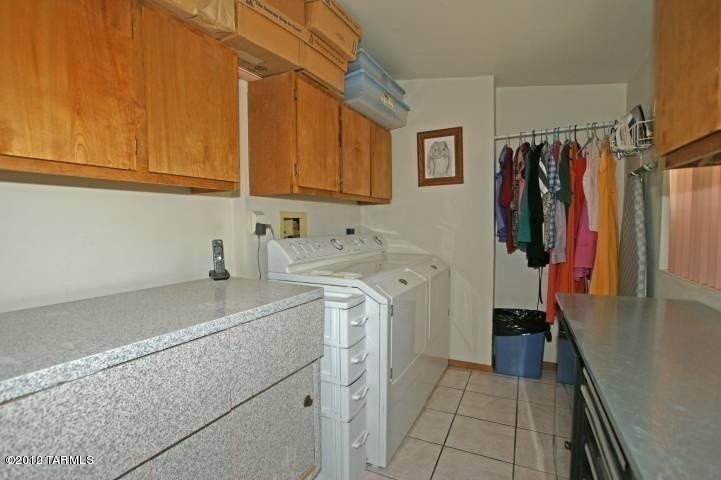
[153,0,409,132]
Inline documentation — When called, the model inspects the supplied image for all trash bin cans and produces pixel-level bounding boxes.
[559,322,579,387]
[493,308,546,378]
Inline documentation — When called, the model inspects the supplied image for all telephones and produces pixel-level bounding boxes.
[211,240,229,281]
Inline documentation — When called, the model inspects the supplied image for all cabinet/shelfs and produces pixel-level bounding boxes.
[321,294,370,480]
[552,302,635,479]
[247,72,340,203]
[341,103,392,206]
[1,1,239,206]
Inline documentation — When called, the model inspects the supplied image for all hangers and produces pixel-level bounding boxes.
[506,121,614,160]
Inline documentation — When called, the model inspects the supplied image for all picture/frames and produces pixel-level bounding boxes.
[417,126,464,187]
